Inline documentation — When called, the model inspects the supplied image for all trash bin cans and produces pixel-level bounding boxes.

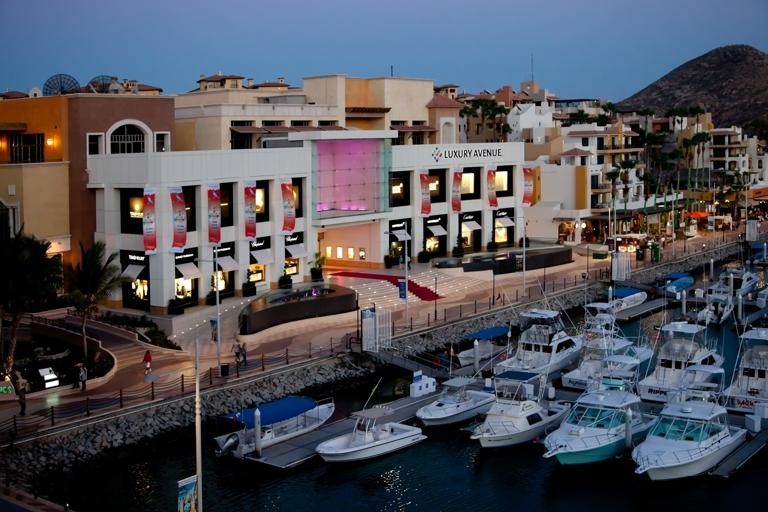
[635,249,644,261]
[221,363,229,378]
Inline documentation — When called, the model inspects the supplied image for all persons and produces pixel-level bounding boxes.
[141,349,153,376]
[79,364,88,391]
[17,383,27,417]
[230,338,240,362]
[238,342,249,369]
[69,366,80,390]
